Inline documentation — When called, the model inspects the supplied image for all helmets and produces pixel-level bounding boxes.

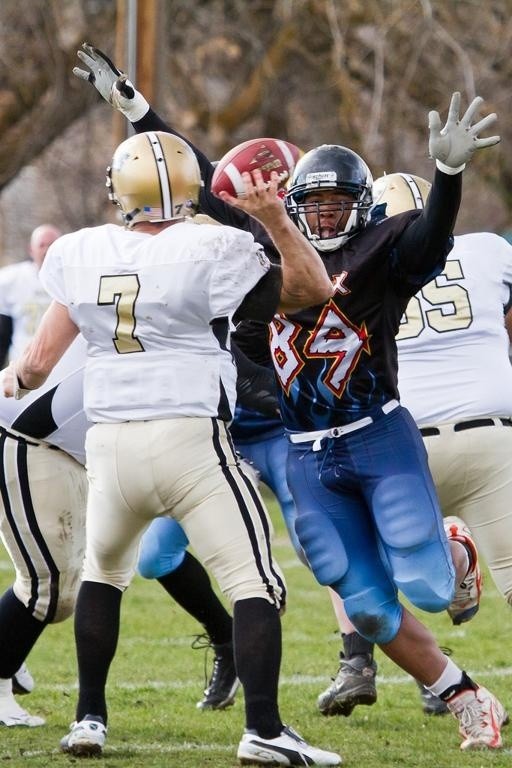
[105,130,203,227]
[360,172,437,232]
[286,144,374,250]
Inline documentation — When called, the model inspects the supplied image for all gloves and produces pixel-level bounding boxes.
[72,41,151,125]
[427,90,502,169]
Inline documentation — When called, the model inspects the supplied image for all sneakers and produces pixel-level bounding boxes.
[1,701,47,728]
[317,648,378,718]
[433,514,484,626]
[196,625,256,713]
[445,668,508,752]
[237,722,344,767]
[59,713,109,753]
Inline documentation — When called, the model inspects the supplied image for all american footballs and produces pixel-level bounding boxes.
[211,138,306,201]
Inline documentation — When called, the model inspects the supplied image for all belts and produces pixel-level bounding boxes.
[418,416,511,440]
[287,400,400,453]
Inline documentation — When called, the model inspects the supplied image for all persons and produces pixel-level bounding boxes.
[3,43,512,766]
